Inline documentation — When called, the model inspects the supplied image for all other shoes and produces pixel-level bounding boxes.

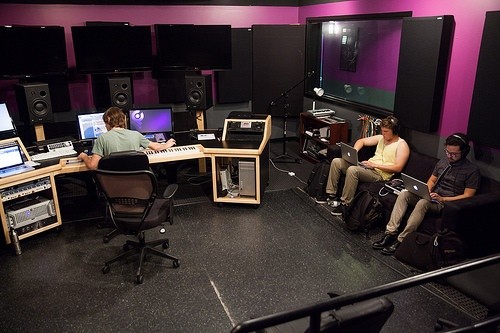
[331,204,347,216]
[316,195,337,204]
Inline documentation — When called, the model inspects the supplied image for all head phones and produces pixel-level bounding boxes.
[390,118,400,134]
[452,134,471,155]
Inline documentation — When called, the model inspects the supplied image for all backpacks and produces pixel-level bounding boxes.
[343,190,382,232]
[305,162,330,200]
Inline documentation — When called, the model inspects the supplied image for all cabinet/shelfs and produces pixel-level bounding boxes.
[298,112,349,165]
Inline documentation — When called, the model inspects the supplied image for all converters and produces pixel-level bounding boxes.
[288,171,295,176]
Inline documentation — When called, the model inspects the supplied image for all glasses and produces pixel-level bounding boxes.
[444,148,461,156]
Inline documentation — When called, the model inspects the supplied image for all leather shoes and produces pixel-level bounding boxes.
[381,240,401,255]
[373,233,397,249]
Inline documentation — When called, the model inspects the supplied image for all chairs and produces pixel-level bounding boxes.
[89,150,180,283]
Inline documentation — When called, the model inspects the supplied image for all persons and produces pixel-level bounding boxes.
[78,107,176,170]
[372,132,481,256]
[314,114,410,215]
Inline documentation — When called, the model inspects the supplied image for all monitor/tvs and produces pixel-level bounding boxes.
[127,107,174,144]
[0,25,70,80]
[75,111,109,147]
[154,24,233,72]
[70,26,152,76]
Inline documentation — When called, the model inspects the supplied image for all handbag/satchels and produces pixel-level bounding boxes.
[395,232,438,271]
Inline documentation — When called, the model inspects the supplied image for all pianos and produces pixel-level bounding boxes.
[140,144,204,163]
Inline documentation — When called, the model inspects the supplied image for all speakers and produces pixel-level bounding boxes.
[467,10,500,148]
[178,74,206,110]
[14,81,54,127]
[394,14,454,133]
[108,76,133,112]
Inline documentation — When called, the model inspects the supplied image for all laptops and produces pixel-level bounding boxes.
[340,142,371,169]
[0,137,42,167]
[0,146,35,178]
[401,173,440,203]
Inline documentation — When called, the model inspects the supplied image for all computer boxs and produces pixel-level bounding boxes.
[238,160,256,196]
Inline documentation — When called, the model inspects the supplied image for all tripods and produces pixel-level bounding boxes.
[269,75,308,160]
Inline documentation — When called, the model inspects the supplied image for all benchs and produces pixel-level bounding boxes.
[327,138,500,263]
